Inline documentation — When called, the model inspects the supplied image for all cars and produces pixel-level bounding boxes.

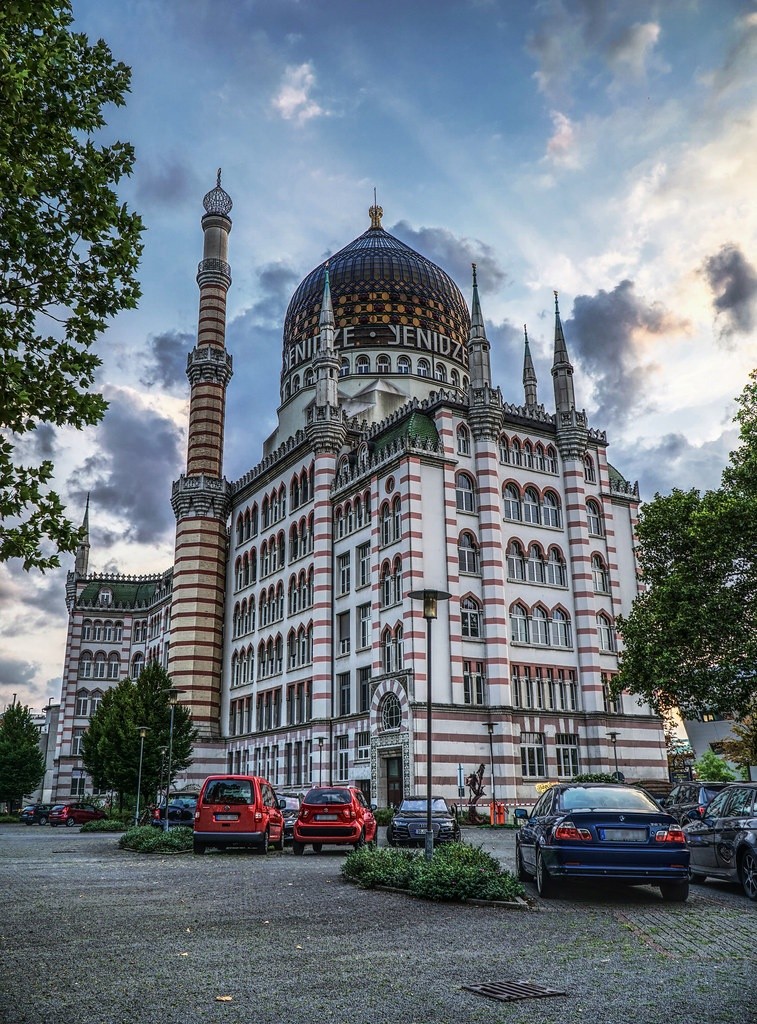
[681,782,757,901]
[19,804,60,826]
[661,781,740,829]
[48,802,108,827]
[513,783,690,903]
[291,786,379,855]
[276,792,305,846]
[387,795,461,848]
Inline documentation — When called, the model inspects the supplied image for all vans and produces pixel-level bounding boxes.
[192,774,286,855]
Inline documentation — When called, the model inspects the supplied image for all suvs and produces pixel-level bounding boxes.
[152,791,200,828]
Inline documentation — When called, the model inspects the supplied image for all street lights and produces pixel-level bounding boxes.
[314,737,328,789]
[606,732,621,783]
[482,722,500,827]
[407,588,454,862]
[134,726,151,826]
[160,688,186,832]
